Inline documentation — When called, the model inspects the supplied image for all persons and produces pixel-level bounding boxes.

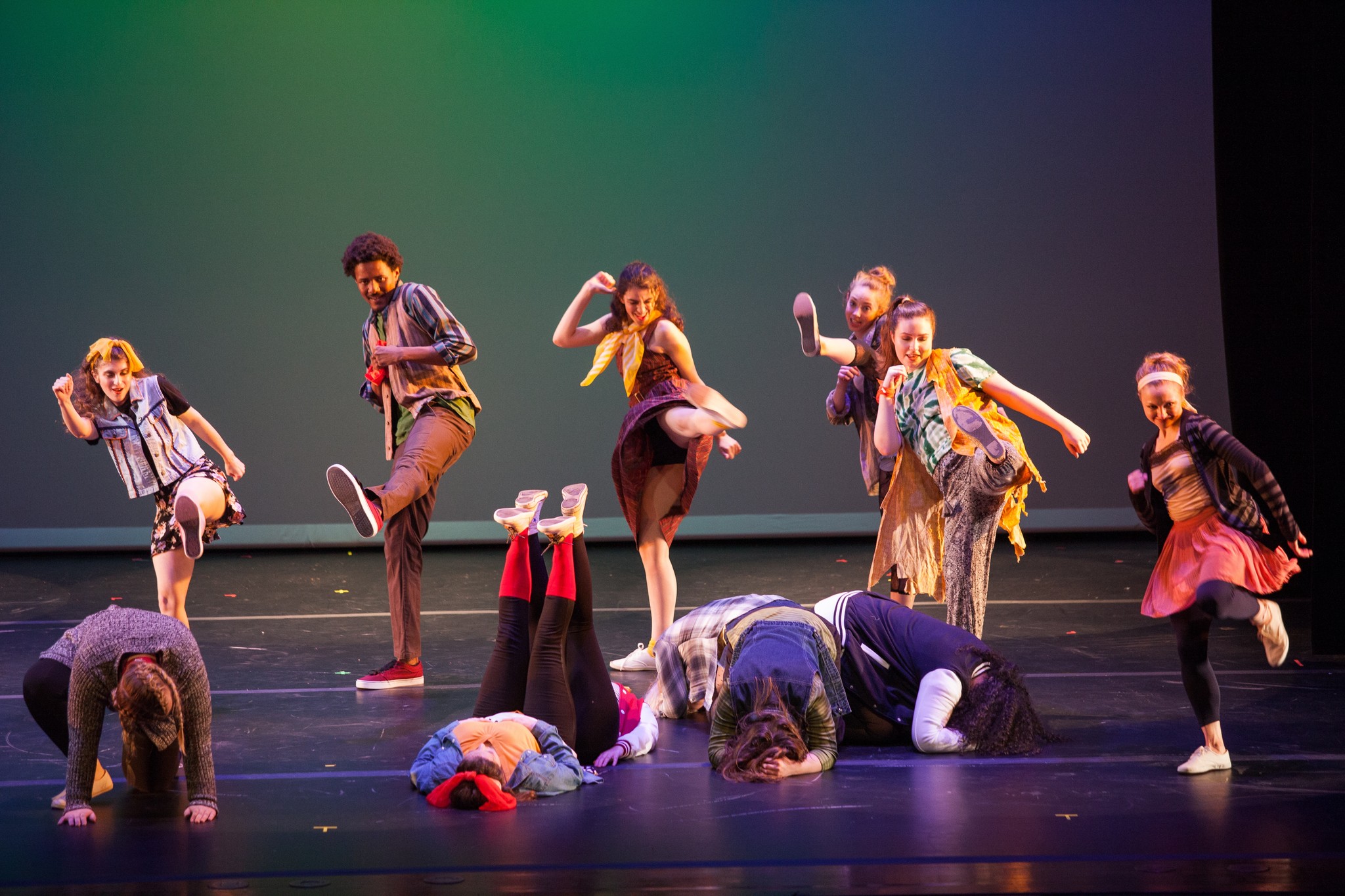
[643,594,804,718]
[23,605,219,827]
[1128,353,1313,774]
[327,232,481,689]
[515,483,659,766]
[867,296,1091,640]
[708,607,852,783]
[553,261,746,670]
[51,338,246,629]
[793,266,915,610]
[411,508,604,811]
[813,590,1058,755]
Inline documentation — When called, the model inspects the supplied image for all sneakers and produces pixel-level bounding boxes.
[326,464,384,538]
[1177,744,1231,774]
[681,382,748,431]
[1253,599,1288,668]
[356,655,424,689]
[610,642,656,673]
[537,516,577,555]
[493,507,534,544]
[561,483,588,520]
[515,487,548,528]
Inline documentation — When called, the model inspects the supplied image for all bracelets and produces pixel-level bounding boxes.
[881,386,895,398]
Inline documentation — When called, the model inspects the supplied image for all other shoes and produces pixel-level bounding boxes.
[793,290,820,356]
[175,495,204,560]
[952,406,1006,461]
[51,768,114,810]
